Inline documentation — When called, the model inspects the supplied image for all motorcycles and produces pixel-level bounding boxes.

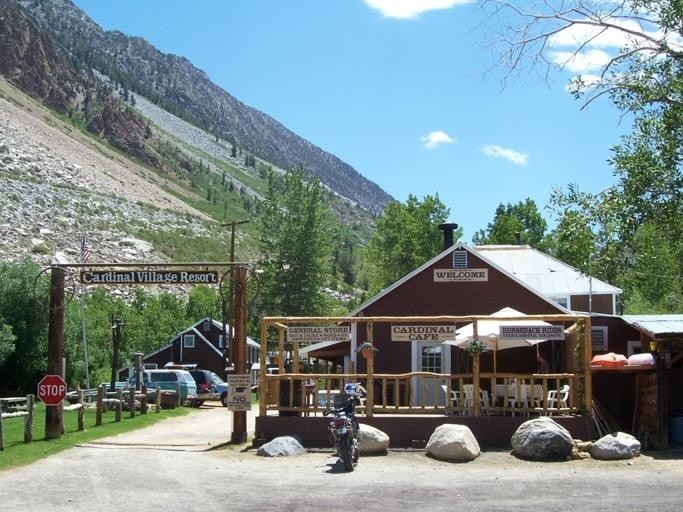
[323,395,363,471]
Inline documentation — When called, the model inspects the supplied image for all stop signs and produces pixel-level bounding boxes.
[36,374,68,407]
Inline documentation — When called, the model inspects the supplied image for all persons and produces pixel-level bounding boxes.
[535,354,550,374]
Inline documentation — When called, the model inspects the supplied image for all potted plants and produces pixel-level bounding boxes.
[464,338,487,357]
[354,340,379,359]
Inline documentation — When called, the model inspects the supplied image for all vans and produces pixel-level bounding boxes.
[126,368,197,408]
[187,365,229,408]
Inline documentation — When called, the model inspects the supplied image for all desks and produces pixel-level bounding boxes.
[490,383,544,416]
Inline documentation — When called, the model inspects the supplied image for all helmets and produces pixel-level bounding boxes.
[344,382,364,397]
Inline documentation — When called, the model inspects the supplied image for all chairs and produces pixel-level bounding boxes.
[510,385,530,420]
[440,383,492,418]
[547,384,572,417]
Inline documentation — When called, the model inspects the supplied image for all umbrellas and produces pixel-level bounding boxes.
[440,306,554,384]
